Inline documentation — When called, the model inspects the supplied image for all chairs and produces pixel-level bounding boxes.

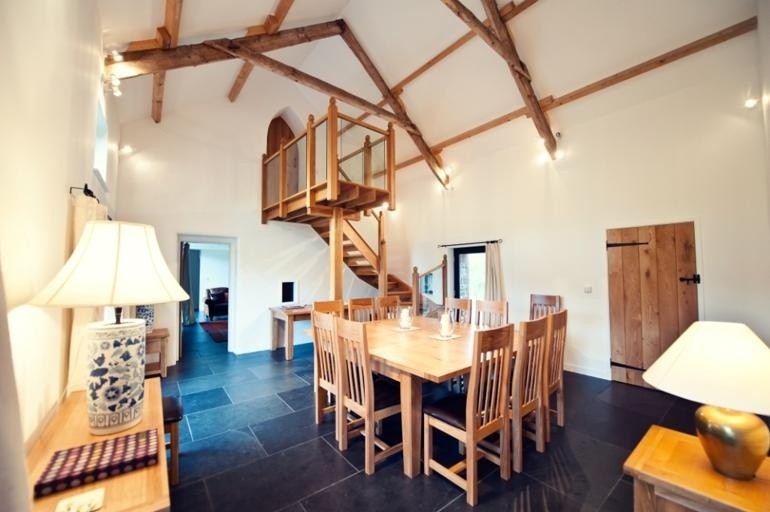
[202,287,227,321]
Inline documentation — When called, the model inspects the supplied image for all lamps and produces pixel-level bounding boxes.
[29,219,191,437]
[641,321,770,482]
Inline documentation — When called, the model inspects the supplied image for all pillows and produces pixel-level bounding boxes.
[208,288,228,303]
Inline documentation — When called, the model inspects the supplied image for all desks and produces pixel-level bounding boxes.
[623,424,770,512]
[25,376,171,511]
[269,303,348,361]
[145,328,170,378]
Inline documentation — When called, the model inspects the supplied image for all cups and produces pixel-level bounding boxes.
[399,308,413,328]
[437,310,454,337]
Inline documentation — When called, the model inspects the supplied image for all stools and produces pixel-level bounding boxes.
[162,396,183,486]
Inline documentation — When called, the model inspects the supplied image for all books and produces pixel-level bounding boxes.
[32,427,158,498]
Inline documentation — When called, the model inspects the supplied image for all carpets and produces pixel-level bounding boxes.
[199,320,227,343]
